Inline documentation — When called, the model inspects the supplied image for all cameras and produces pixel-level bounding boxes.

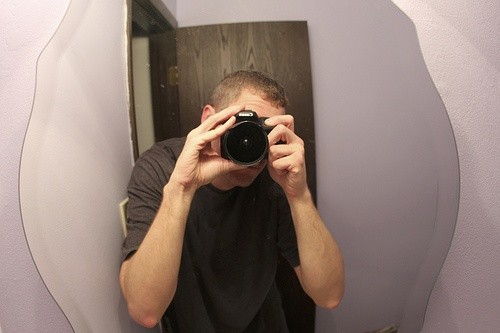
[221,109,285,167]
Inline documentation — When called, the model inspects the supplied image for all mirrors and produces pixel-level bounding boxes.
[17,1,461,333]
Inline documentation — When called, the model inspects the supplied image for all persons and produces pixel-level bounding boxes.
[116,69,345,332]
